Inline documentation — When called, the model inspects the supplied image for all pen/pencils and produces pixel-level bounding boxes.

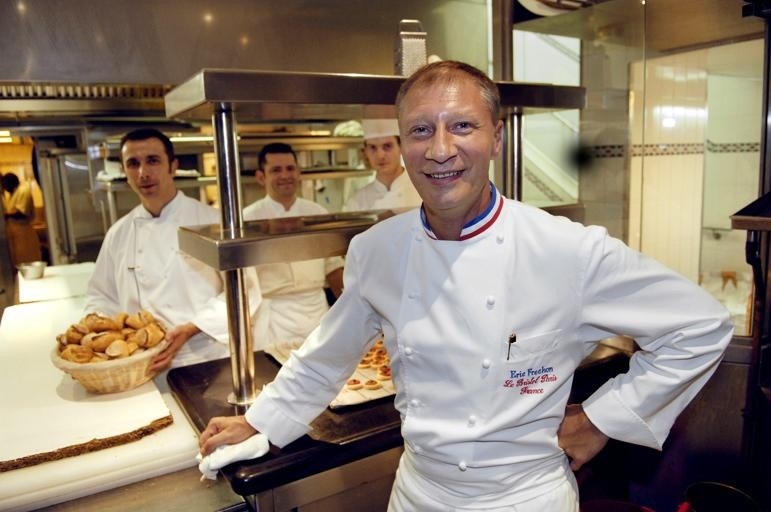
[507,333,517,361]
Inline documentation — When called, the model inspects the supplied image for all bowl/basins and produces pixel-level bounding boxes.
[14,261,47,279]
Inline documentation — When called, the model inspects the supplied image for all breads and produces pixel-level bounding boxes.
[54,313,166,365]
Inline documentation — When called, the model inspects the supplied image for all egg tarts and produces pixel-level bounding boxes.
[346,336,392,390]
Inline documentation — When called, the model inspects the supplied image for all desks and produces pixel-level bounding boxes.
[2,298,246,511]
[13,261,98,303]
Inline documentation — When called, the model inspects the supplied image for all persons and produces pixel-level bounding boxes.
[84,127,262,378]
[340,119,424,216]
[199,61,734,512]
[1,171,41,270]
[242,143,343,364]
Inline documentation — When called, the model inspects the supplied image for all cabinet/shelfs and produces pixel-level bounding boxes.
[88,131,374,246]
[163,66,589,394]
[164,341,628,511]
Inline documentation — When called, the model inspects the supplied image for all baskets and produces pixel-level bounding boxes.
[50,338,172,395]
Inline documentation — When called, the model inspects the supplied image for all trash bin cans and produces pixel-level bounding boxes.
[676,480,761,512]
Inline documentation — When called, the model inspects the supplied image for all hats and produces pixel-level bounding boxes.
[361,119,400,141]
